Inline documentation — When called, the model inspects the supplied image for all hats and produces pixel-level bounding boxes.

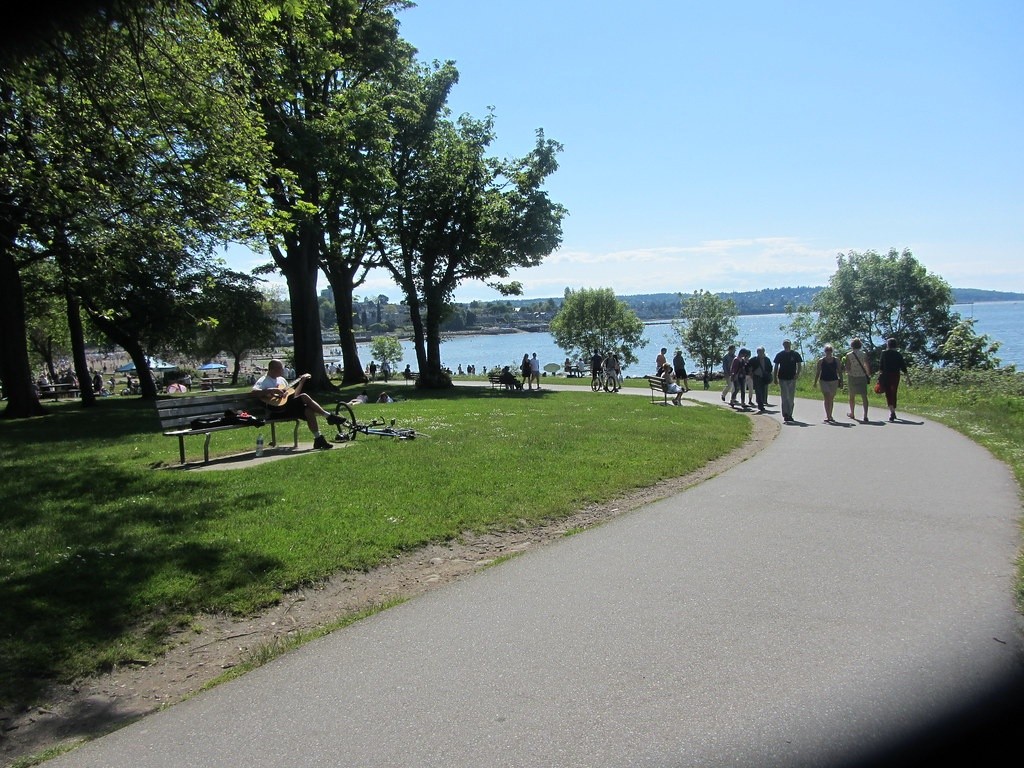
[783,338,790,344]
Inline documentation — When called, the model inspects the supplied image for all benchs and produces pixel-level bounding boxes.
[38,389,81,399]
[487,372,517,391]
[648,375,671,407]
[570,369,590,378]
[401,372,420,386]
[154,392,300,467]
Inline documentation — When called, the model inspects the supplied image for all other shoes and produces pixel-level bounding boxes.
[847,412,854,419]
[890,412,897,423]
[721,393,769,411]
[824,417,835,422]
[864,418,868,422]
[686,388,691,390]
[618,386,621,389]
[326,413,347,425]
[673,399,682,407]
[314,434,333,449]
[784,415,793,421]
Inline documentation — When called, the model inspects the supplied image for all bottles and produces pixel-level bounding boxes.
[256,434,264,457]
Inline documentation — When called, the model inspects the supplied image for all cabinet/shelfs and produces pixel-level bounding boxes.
[200,382,228,386]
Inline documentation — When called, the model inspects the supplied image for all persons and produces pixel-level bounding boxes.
[32,359,82,399]
[376,392,394,403]
[166,371,209,393]
[656,348,691,405]
[247,375,256,385]
[325,362,341,374]
[812,345,843,423]
[447,363,475,374]
[878,338,912,423]
[370,361,376,383]
[522,353,541,389]
[721,345,773,411]
[251,359,347,449]
[499,366,520,389]
[591,349,621,392]
[284,364,295,378]
[380,360,390,382]
[86,356,138,397]
[565,358,584,377]
[347,390,368,405]
[405,364,410,378]
[773,339,803,423]
[845,339,870,423]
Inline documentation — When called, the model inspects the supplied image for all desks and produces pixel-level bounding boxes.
[40,383,75,402]
[571,366,587,377]
[202,377,224,392]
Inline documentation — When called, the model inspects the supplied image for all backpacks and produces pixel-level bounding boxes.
[224,408,266,428]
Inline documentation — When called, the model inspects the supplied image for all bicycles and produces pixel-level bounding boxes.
[591,366,616,393]
[334,402,431,440]
[121,388,142,395]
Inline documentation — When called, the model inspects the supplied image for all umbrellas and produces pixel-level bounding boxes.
[198,362,227,370]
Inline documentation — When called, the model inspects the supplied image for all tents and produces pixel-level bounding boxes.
[115,355,175,371]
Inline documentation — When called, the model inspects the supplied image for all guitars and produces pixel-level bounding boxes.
[257,373,311,411]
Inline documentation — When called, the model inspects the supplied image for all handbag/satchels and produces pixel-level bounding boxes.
[837,378,843,387]
[762,372,773,384]
[190,416,223,430]
[617,372,623,384]
[866,377,872,385]
[874,377,884,394]
